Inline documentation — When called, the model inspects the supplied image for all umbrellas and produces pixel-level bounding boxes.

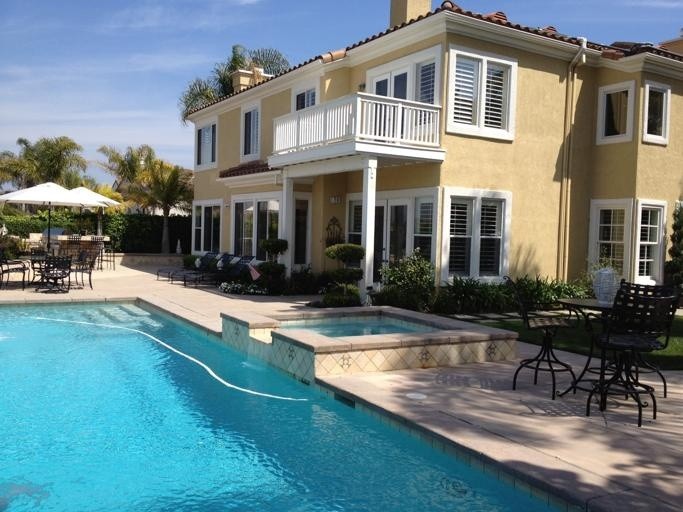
[69,186,123,236]
[0,182,108,251]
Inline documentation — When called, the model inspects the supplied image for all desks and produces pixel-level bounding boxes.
[556,295,652,406]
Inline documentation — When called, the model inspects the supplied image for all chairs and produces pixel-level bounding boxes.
[606,278,677,397]
[189,256,253,290]
[502,274,578,398]
[155,251,218,283]
[587,289,674,427]
[0,231,114,294]
[171,252,235,285]
